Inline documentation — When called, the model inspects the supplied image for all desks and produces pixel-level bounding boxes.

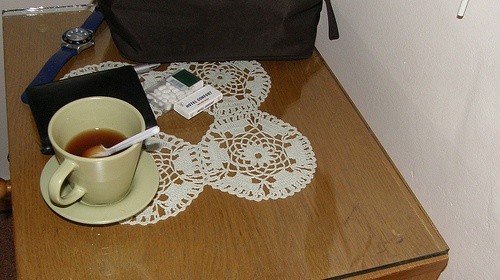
[2,10,451,280]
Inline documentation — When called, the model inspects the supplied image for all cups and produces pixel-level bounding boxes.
[48,96,146,207]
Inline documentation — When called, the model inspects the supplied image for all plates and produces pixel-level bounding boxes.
[39,149,160,224]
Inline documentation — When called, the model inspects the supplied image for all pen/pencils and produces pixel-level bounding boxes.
[133,63,161,73]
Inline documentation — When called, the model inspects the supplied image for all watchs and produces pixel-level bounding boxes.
[21,7,106,105]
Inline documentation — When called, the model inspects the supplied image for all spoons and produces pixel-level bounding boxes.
[81,126,160,158]
